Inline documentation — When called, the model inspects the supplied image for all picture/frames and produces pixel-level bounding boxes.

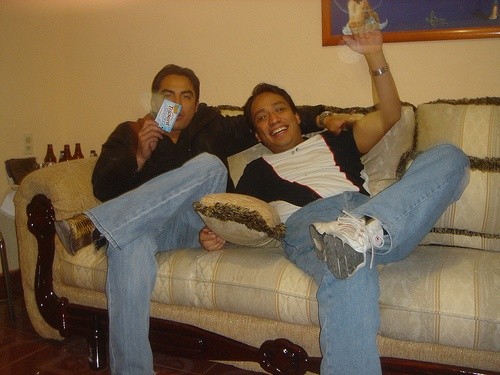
[321,0,500,46]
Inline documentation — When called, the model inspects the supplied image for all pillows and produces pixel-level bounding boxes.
[192,192,287,249]
[396,149,500,239]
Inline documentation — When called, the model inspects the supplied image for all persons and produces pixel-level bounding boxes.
[54,65,365,375]
[181,0,470,375]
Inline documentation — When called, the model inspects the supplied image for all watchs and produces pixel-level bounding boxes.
[319,111,332,127]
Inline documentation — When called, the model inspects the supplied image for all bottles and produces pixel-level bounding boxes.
[72,143,84,160]
[43,144,57,168]
[85,312,106,369]
[89,150,97,158]
[58,144,72,163]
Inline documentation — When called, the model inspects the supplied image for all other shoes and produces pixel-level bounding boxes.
[53,210,96,255]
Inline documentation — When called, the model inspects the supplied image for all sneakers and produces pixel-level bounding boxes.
[308,210,386,280]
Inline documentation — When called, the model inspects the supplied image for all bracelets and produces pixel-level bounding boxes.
[370,63,390,77]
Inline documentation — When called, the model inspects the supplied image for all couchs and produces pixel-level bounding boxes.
[13,97,500,375]
